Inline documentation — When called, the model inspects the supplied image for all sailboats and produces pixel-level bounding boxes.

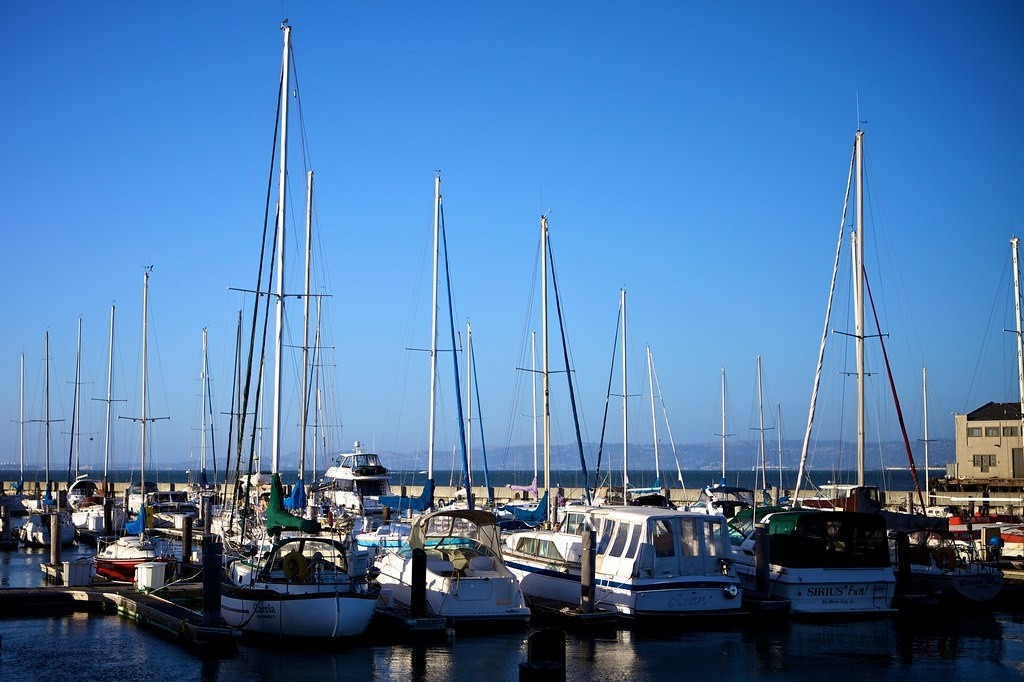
[2,22,1024,653]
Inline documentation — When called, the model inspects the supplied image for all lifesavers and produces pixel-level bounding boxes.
[282,552,308,580]
[935,547,955,569]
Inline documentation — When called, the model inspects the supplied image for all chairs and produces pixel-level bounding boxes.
[464,557,492,577]
